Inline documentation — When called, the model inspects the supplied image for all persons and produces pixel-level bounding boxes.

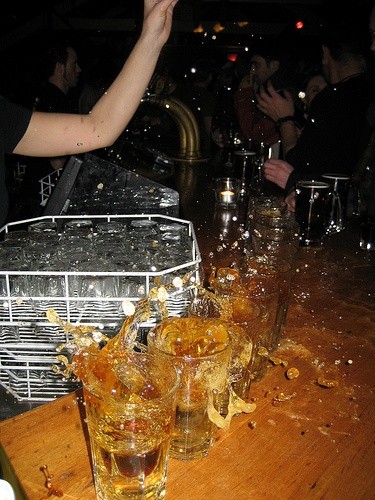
[256,17,375,175]
[79,38,333,199]
[0,0,179,226]
[264,7,375,218]
[34,42,80,217]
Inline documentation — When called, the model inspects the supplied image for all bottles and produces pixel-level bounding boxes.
[222,130,238,177]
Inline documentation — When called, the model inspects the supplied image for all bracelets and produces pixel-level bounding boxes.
[273,115,297,134]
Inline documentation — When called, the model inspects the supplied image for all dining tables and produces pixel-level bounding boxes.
[0,212,374,499]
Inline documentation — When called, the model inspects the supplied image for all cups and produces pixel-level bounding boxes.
[234,151,261,189]
[239,255,293,325]
[296,180,343,247]
[215,274,280,382]
[81,353,180,500]
[320,174,361,237]
[1,220,193,300]
[248,196,299,265]
[188,295,262,405]
[147,317,232,460]
[215,177,241,210]
